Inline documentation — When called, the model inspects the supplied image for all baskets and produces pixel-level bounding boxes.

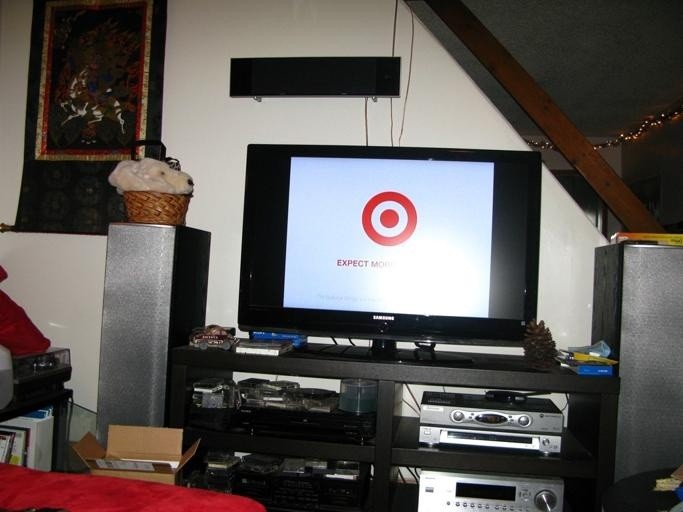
[123,190,193,226]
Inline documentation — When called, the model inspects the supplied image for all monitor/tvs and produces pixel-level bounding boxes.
[237,142,542,365]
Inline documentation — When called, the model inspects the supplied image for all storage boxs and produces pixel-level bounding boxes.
[70,424,203,487]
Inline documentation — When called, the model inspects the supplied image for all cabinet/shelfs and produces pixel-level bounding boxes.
[169,336,621,512]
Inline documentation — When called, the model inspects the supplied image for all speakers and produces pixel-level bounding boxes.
[590,240,683,512]
[94,221,211,446]
